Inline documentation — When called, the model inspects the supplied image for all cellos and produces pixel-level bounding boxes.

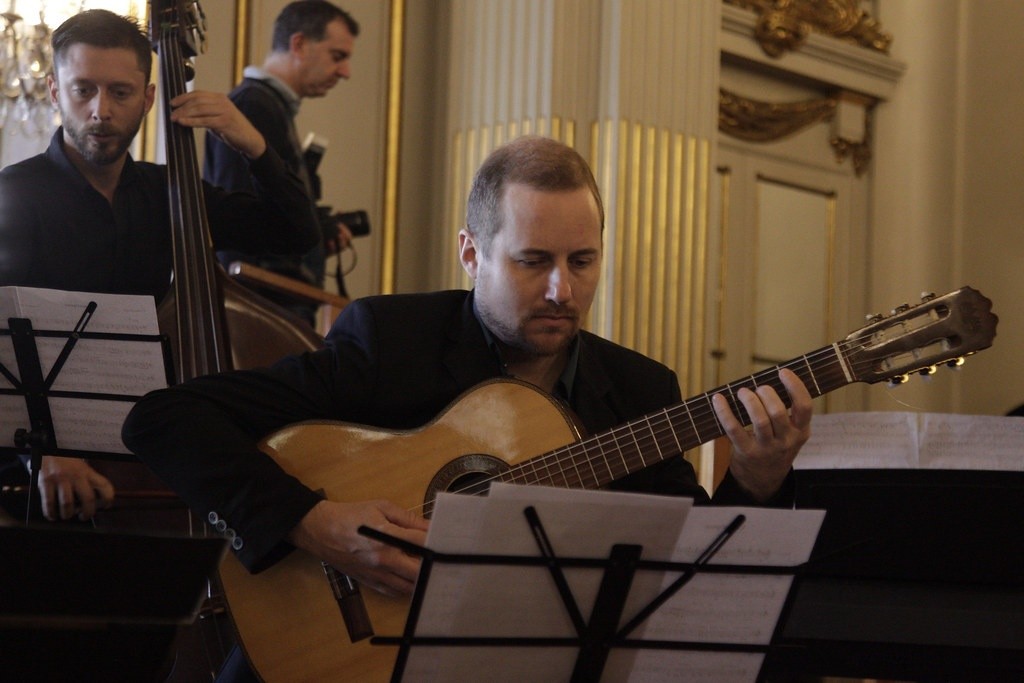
[81,0,326,683]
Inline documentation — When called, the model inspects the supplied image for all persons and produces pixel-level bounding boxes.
[202,0,358,338]
[119,134,817,683]
[0,9,326,528]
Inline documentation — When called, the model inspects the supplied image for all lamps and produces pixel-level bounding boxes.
[0,0,60,138]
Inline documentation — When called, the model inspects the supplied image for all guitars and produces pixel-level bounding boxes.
[218,282,1001,683]
